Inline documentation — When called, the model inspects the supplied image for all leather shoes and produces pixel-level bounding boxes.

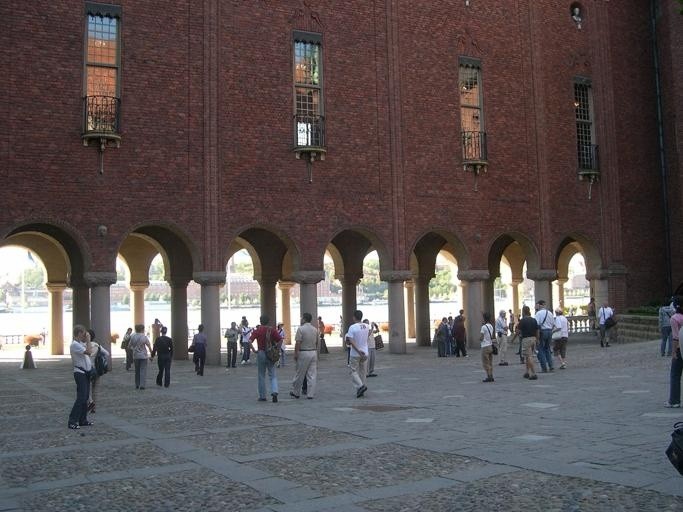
[68,423,81,429]
[80,421,94,426]
[272,393,278,402]
[290,391,299,398]
[483,376,494,382]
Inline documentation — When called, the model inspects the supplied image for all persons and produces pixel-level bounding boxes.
[479,300,573,382]
[572,8,582,22]
[597,304,614,347]
[659,294,683,408]
[345,310,380,398]
[434,309,466,357]
[68,314,325,429]
[588,298,596,329]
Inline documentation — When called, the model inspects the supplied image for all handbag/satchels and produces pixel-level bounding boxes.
[189,346,194,352]
[665,421,682,476]
[375,332,384,350]
[86,368,97,380]
[491,344,498,355]
[551,329,561,340]
[604,318,617,330]
[95,346,109,376]
[265,327,281,362]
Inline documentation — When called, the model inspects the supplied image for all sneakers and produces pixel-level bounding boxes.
[241,359,246,365]
[247,359,251,365]
[523,362,568,380]
[88,402,96,412]
[663,400,679,408]
[356,384,367,398]
[91,409,96,413]
